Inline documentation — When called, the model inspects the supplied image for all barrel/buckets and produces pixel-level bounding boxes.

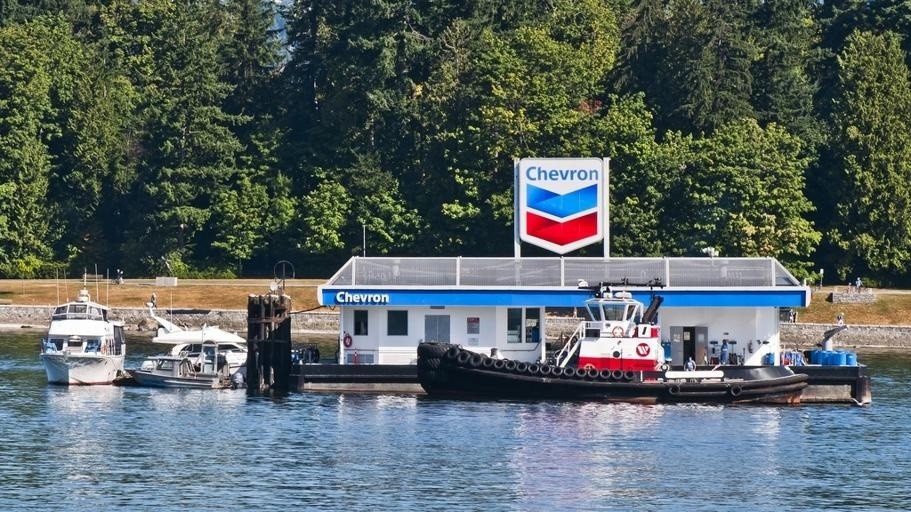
[812,349,856,366]
[765,353,774,365]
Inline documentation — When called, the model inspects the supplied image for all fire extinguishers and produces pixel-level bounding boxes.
[353,351,358,365]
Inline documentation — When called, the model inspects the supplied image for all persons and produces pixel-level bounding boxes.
[856,277,863,291]
[721,338,729,364]
[150,293,157,309]
[682,355,696,372]
[787,309,795,323]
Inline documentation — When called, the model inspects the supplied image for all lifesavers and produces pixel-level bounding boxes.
[100,343,108,352]
[39,345,45,352]
[585,363,594,368]
[344,336,352,347]
[612,326,623,337]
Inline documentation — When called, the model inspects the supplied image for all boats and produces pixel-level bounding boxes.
[408,278,809,409]
[41,263,252,393]
